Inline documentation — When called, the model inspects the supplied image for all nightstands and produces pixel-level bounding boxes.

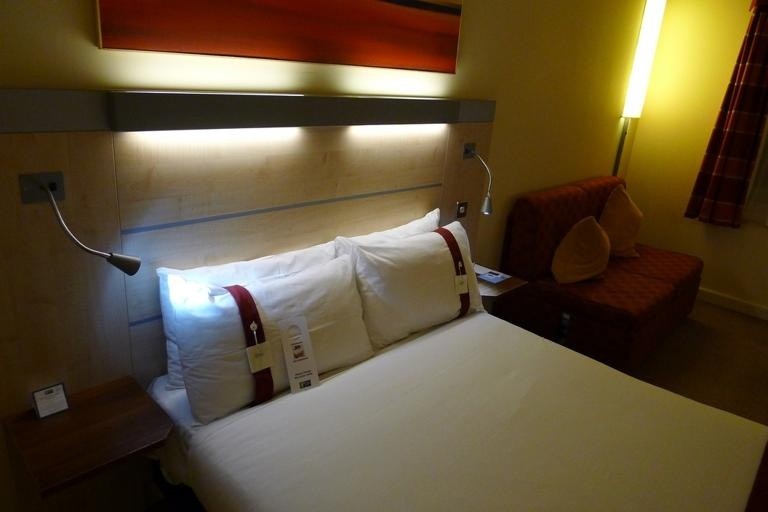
[477,273,528,327]
[4,375,174,512]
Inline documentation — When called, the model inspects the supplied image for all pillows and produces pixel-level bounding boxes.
[172,253,374,427]
[551,216,610,286]
[351,221,486,353]
[598,184,644,258]
[156,240,336,391]
[336,207,440,258]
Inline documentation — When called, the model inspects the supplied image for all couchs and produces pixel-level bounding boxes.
[498,174,705,372]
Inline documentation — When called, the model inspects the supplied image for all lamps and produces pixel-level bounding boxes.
[19,171,141,277]
[613,0,668,175]
[463,142,493,216]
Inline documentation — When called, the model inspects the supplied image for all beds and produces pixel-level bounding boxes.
[144,313,768,512]
